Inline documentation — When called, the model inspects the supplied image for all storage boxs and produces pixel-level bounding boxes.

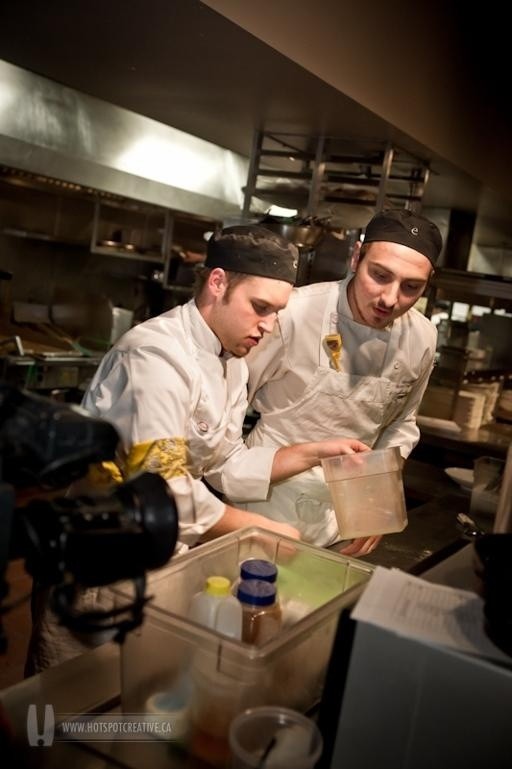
[96,525,378,768]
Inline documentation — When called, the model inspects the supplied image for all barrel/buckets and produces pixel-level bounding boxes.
[187,576,243,647]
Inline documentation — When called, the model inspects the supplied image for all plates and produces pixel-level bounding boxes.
[444,467,476,492]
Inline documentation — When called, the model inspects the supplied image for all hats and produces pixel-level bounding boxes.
[363,208,442,268]
[205,225,298,284]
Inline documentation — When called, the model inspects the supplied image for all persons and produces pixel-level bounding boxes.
[23,222,373,683]
[223,208,443,560]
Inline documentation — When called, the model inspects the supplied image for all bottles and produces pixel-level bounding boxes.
[187,559,283,647]
